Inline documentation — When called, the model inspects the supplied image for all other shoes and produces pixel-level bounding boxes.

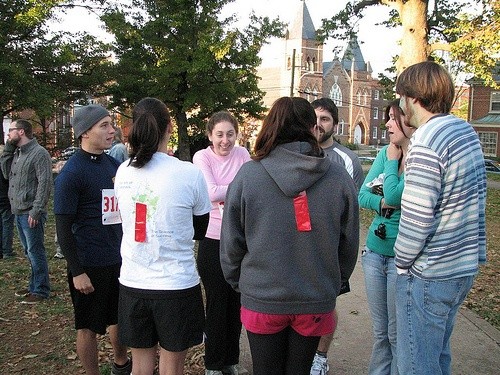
[226,364,249,375]
[54,252,65,259]
[1,252,17,259]
[110,356,132,375]
[20,294,44,304]
[14,288,31,297]
[204,369,223,375]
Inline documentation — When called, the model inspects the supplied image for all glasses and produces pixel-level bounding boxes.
[9,127,24,133]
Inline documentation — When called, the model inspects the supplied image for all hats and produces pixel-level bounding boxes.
[73,104,111,139]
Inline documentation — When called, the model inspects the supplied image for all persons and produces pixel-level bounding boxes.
[310,98,366,375]
[219,97,359,375]
[357,99,417,375]
[114,97,214,375]
[108,126,129,163]
[191,111,251,375]
[52,104,132,375]
[392,61,487,375]
[0,119,53,304]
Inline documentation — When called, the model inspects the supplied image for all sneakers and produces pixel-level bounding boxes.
[310,352,330,375]
